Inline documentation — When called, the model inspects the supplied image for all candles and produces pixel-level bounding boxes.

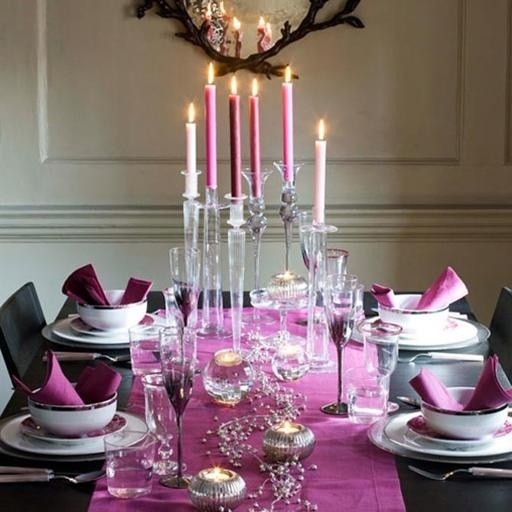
[184,102,198,193]
[313,120,324,226]
[280,65,294,180]
[202,61,218,188]
[248,78,260,196]
[227,74,241,197]
[205,0,272,60]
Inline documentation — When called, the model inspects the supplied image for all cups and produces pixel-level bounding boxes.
[103,246,401,497]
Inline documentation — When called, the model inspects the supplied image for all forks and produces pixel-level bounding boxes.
[408,464,512,483]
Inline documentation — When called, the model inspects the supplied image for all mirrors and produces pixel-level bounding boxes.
[134,1,363,79]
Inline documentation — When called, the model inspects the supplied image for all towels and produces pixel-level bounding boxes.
[409,354,512,413]
[14,348,122,405]
[61,263,152,305]
[369,266,469,312]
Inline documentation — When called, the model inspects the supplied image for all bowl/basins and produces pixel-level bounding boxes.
[377,293,449,334]
[25,389,118,437]
[74,298,148,331]
[419,386,508,440]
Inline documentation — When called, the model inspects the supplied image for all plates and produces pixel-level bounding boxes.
[0,416,149,457]
[41,317,170,352]
[367,411,512,457]
[350,317,490,349]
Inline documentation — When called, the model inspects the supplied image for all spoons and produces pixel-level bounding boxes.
[0,469,106,484]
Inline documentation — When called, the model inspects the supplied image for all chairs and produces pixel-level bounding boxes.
[1,282,50,388]
[484,286,512,385]
